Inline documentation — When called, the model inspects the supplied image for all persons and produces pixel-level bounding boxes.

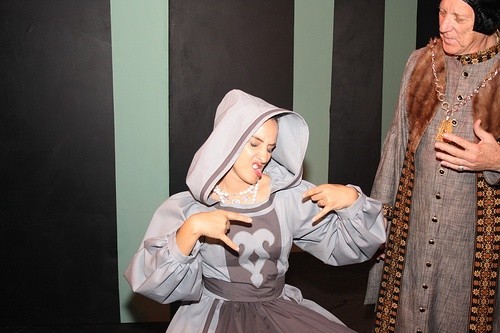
[362,0,500,333]
[122,89,388,332]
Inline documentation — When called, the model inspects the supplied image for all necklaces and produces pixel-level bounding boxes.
[212,180,259,205]
[429,36,500,141]
